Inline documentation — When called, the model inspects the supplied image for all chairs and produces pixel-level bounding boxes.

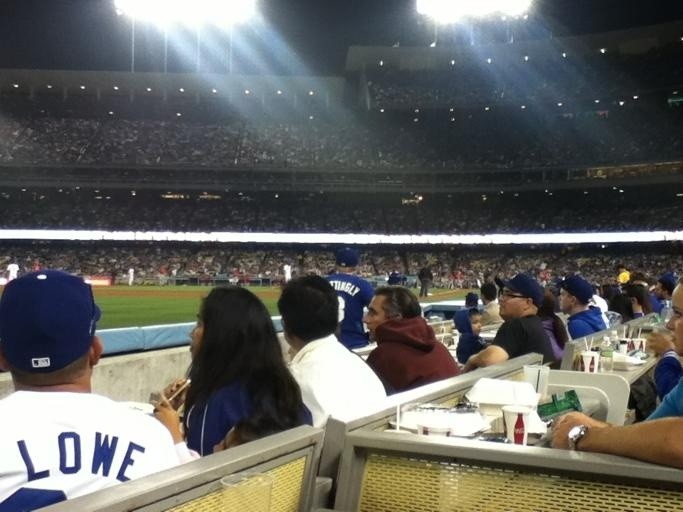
[1,111,683,166]
[0,245,683,512]
[374,78,683,108]
[0,194,683,231]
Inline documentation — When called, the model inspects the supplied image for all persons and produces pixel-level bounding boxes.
[554,278,683,469]
[0,269,180,512]
[1,71,683,297]
[323,248,375,351]
[275,274,386,426]
[455,271,677,370]
[363,286,462,396]
[155,285,313,467]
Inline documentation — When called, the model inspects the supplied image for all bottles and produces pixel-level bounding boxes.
[600,336,613,373]
[610,329,618,350]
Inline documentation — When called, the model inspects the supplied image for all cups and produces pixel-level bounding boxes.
[631,338,646,352]
[620,340,626,352]
[621,337,631,352]
[524,364,549,400]
[218,472,273,511]
[578,351,600,372]
[500,406,532,444]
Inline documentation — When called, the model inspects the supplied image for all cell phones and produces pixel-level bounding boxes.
[152,377,192,413]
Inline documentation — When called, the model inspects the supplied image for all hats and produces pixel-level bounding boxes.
[556,277,592,303]
[336,247,358,266]
[1,269,101,372]
[494,275,544,307]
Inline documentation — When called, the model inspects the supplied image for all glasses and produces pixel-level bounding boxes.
[498,290,525,299]
